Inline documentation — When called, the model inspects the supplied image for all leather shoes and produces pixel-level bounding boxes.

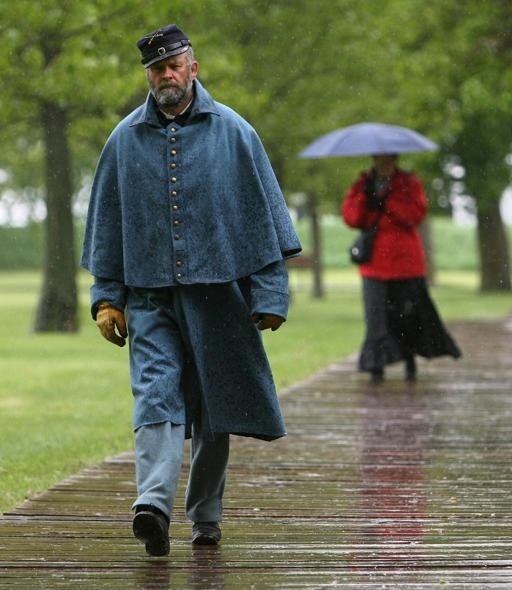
[192,521,220,545]
[133,512,169,556]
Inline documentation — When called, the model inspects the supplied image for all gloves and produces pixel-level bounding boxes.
[97,302,127,347]
[252,314,284,331]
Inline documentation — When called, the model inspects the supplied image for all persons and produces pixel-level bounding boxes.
[80,23,305,558]
[340,153,463,380]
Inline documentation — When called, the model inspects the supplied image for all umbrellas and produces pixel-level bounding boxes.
[298,123,441,159]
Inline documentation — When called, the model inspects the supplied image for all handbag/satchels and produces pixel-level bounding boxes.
[351,233,374,262]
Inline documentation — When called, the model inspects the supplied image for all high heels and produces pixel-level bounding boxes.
[373,368,383,380]
[407,361,415,380]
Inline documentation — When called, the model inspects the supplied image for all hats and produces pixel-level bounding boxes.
[137,24,192,69]
